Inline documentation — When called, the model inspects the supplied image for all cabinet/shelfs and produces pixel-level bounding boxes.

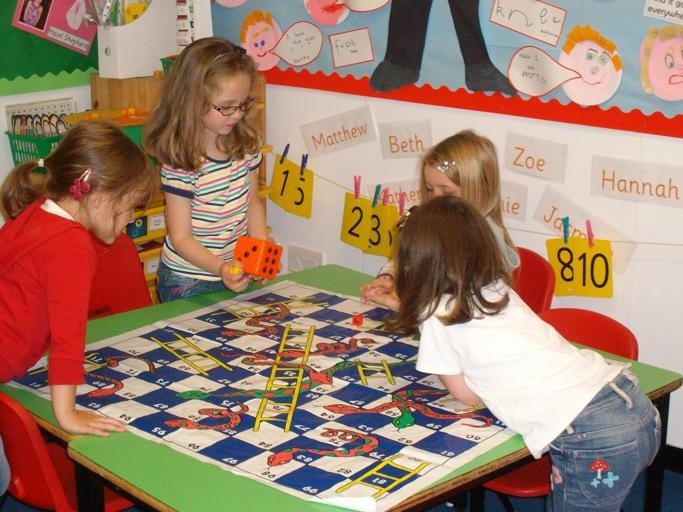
[74,70,270,307]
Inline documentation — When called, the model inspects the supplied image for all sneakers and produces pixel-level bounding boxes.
[368,59,421,93]
[464,72,518,97]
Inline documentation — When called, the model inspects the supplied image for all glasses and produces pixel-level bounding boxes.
[208,99,255,116]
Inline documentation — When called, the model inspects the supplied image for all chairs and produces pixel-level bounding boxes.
[1,393,137,512]
[509,245,558,314]
[87,229,155,324]
[483,307,640,512]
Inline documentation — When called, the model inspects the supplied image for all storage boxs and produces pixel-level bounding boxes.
[120,204,169,244]
[138,246,163,279]
[147,280,161,307]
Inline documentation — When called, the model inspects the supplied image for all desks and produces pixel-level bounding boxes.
[1,262,683,512]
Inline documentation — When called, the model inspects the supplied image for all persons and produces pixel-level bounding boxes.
[0,118,157,500]
[22,0,43,26]
[140,36,283,304]
[381,193,664,512]
[355,128,522,314]
